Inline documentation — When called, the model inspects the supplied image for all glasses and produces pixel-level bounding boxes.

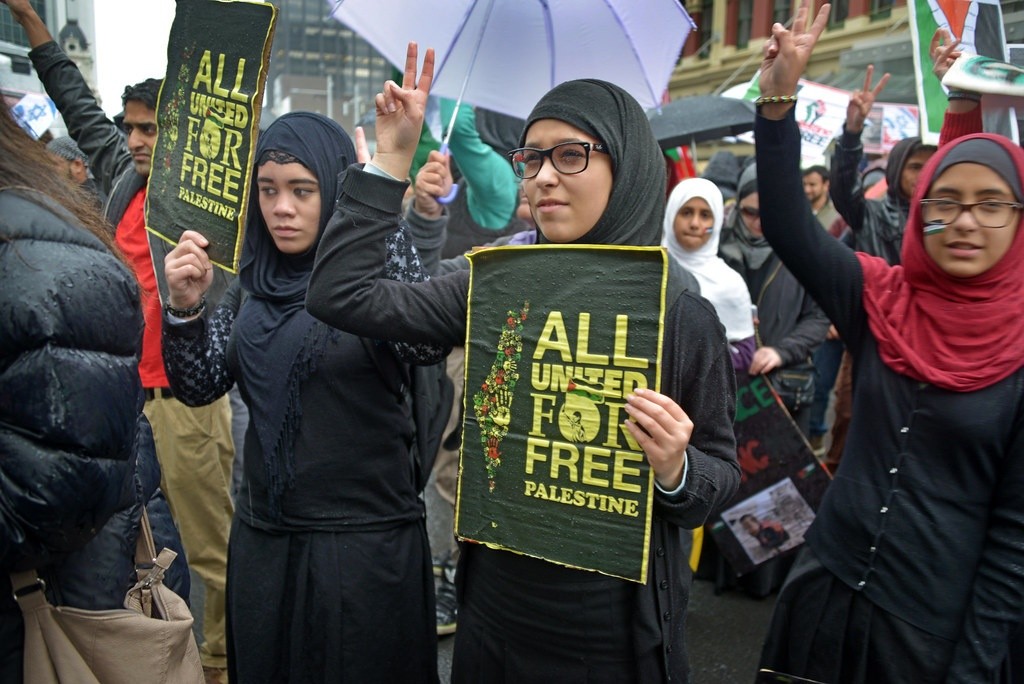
[508,141,611,179]
[920,198,1024,228]
[738,205,761,217]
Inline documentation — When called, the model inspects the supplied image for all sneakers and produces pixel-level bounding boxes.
[434,561,458,634]
[432,556,441,575]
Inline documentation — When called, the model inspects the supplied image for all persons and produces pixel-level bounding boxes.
[1,0,249,684]
[663,25,985,600]
[739,514,791,549]
[402,99,535,632]
[163,111,440,684]
[0,92,145,684]
[302,43,741,684]
[754,1,1023,684]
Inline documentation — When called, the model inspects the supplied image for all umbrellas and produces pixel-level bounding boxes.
[650,94,758,174]
[327,0,698,203]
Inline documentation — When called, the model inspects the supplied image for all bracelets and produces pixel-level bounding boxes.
[948,91,983,102]
[166,296,205,317]
[757,94,798,106]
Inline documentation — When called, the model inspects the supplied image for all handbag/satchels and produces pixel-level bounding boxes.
[768,364,820,411]
[22,581,206,684]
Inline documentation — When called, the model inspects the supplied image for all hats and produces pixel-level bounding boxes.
[45,136,88,166]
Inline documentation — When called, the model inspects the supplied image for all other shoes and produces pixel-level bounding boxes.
[202,665,228,684]
